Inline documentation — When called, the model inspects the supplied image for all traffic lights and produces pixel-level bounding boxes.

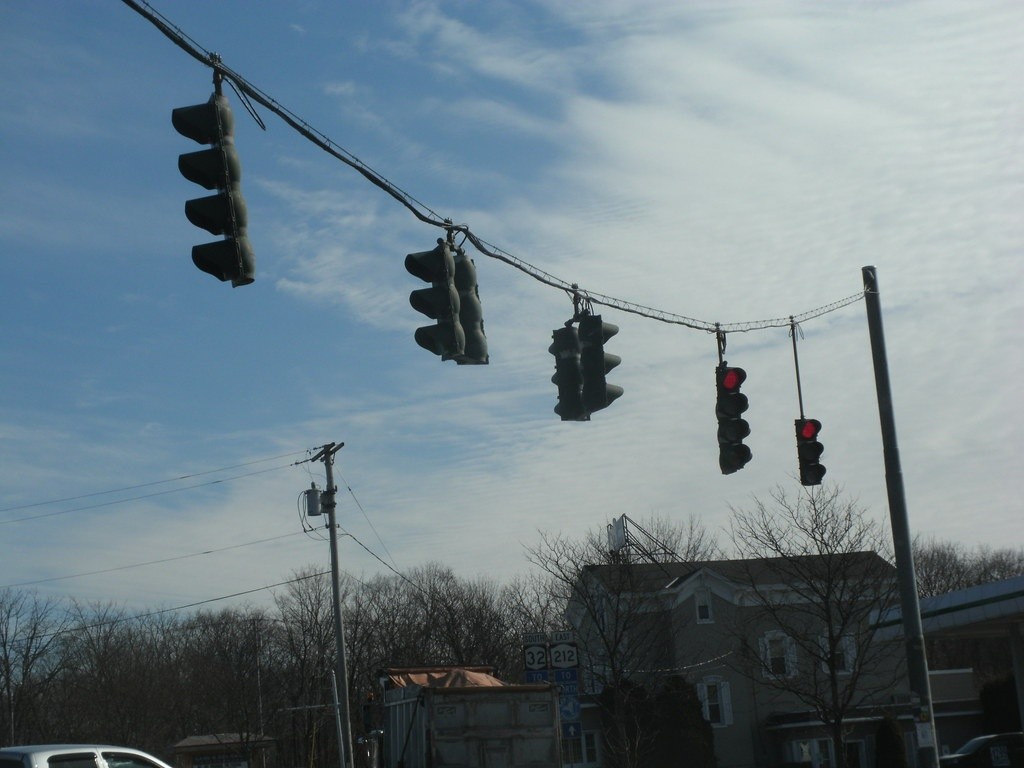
[715,361,753,475]
[172,95,256,287]
[582,315,624,413]
[794,418,827,485]
[404,238,465,361]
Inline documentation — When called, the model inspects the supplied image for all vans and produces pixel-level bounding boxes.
[0,743,174,768]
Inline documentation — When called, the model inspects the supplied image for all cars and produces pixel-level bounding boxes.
[939,731,1024,768]
